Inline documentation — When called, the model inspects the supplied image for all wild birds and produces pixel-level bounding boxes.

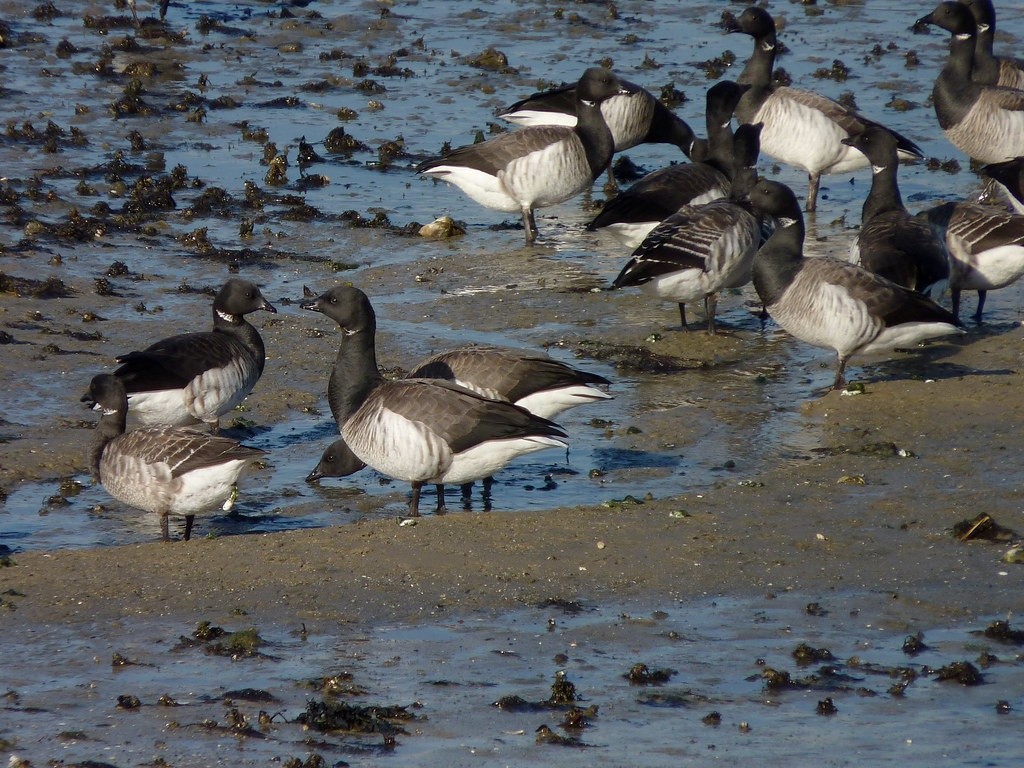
[307,350,614,495]
[87,280,277,429]
[914,201,1024,321]
[494,79,700,190]
[916,0,1024,214]
[300,286,569,516]
[726,178,968,390]
[724,6,926,213]
[583,81,777,337]
[80,374,270,541]
[841,127,955,304]
[415,67,633,243]
